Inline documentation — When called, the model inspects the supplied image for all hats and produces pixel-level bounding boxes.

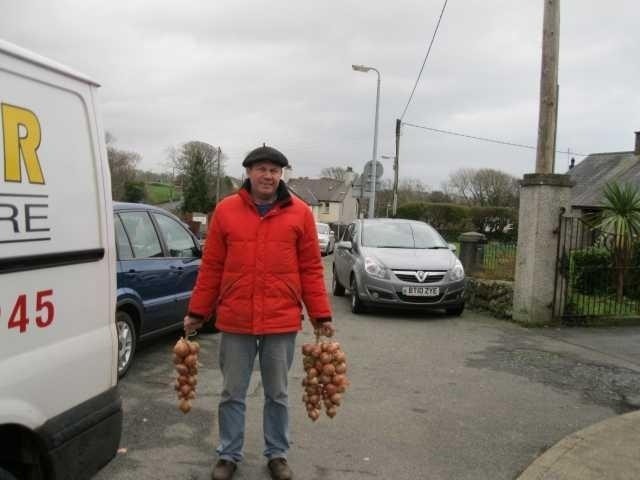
[243,143,287,167]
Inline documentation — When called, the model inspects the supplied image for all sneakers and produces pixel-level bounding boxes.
[268,457,291,480]
[212,458,237,480]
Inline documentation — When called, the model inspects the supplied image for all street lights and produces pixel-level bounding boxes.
[382,153,399,217]
[351,63,383,217]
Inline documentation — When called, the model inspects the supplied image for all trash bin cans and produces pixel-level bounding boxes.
[458,231,488,278]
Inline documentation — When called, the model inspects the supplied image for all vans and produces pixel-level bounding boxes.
[0,36,136,480]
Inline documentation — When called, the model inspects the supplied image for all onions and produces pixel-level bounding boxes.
[173,338,200,411]
[301,342,350,421]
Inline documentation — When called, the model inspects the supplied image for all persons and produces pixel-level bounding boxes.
[184,145,336,480]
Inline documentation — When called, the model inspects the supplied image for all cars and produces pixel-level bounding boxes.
[113,201,221,381]
[329,216,466,316]
[315,220,337,257]
